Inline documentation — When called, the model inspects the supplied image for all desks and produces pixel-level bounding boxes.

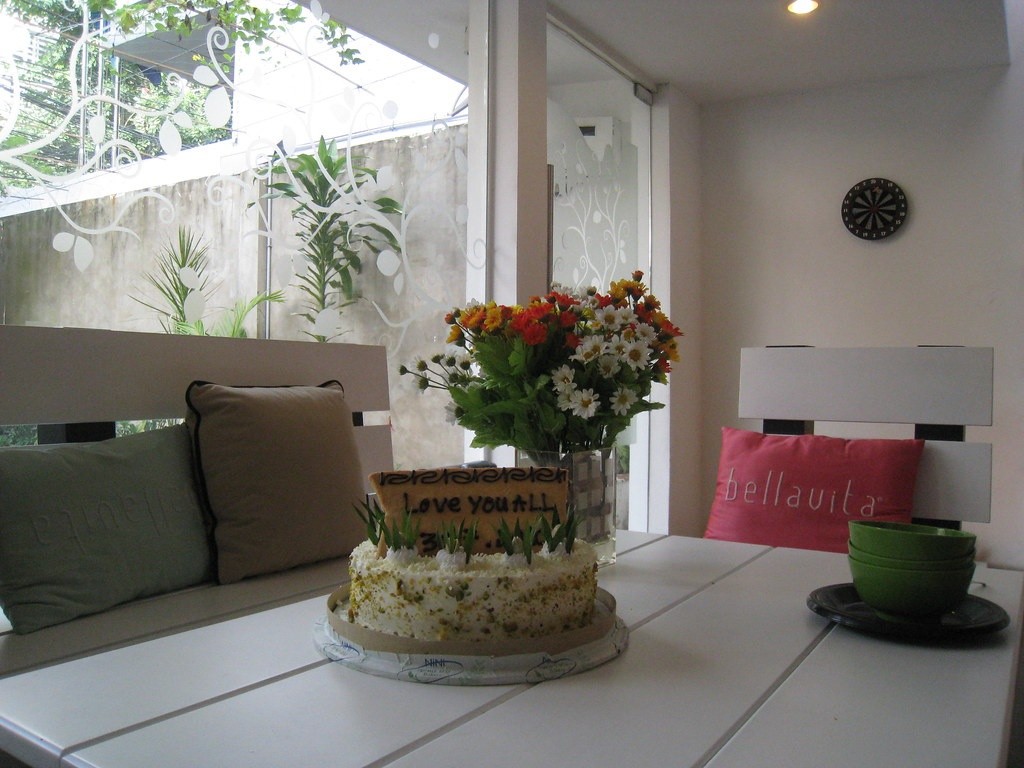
[0,531,1024,768]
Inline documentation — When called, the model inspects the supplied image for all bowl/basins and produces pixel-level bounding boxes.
[847,518,978,622]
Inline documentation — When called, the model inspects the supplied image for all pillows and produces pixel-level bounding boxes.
[704,427,923,556]
[0,424,214,635]
[185,379,372,585]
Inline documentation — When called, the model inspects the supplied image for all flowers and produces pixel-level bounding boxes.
[400,269,682,465]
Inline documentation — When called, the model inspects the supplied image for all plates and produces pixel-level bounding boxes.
[806,582,1010,647]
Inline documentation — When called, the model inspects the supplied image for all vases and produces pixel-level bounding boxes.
[514,445,617,566]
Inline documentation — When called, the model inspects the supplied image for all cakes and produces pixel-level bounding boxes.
[345,465,599,643]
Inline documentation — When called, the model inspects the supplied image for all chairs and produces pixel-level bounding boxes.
[738,345,993,532]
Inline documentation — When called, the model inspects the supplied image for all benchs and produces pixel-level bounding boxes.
[0,324,393,681]
